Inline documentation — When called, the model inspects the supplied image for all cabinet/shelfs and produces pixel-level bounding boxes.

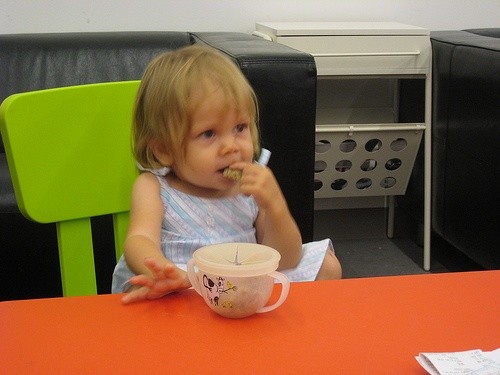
[254,22,432,273]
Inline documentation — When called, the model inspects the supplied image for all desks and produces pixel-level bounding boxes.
[0,268,500,375]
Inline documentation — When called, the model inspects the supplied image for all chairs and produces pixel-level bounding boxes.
[1,79,145,298]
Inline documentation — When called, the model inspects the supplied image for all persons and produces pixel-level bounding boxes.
[111,43,343,305]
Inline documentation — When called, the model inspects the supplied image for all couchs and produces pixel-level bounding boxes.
[387,27,500,271]
[0,32,317,307]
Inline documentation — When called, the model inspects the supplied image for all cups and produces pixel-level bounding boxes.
[188,242,290,319]
